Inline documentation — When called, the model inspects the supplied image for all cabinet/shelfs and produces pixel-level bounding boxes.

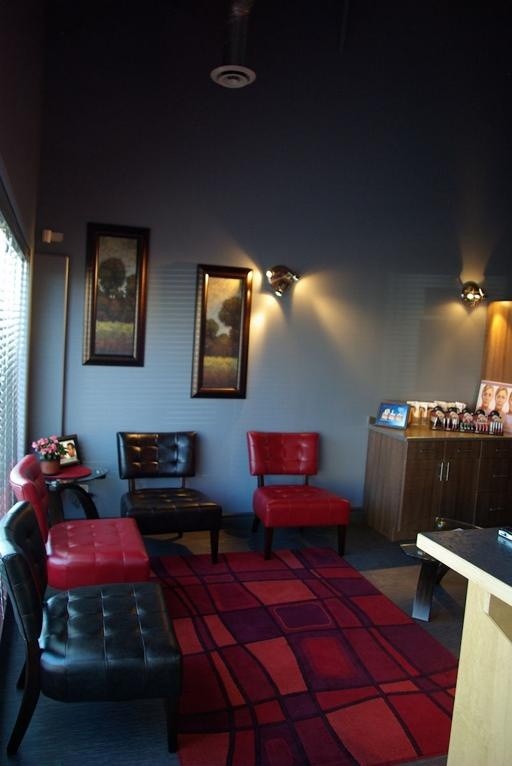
[361,416,512,542]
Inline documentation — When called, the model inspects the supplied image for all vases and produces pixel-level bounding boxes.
[40,460,60,475]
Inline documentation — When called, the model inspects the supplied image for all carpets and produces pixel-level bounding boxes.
[158,546,460,765]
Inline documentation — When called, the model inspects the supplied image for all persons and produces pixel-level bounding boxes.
[477,384,494,416]
[494,386,508,423]
[67,443,76,461]
[504,392,512,433]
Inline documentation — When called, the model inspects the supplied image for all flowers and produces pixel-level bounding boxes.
[31,434,68,461]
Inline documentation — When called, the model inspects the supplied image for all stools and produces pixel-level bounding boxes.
[401,514,482,622]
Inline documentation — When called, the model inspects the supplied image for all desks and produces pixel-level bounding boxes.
[40,463,109,525]
[410,523,512,766]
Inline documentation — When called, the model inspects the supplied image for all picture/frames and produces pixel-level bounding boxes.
[472,379,512,434]
[192,263,248,402]
[374,397,410,430]
[55,434,83,469]
[80,220,150,369]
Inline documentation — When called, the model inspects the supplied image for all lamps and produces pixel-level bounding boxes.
[266,265,298,296]
[459,280,484,310]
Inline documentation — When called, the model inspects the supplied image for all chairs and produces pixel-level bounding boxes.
[1,500,180,761]
[10,453,152,592]
[246,430,352,561]
[116,429,223,564]
